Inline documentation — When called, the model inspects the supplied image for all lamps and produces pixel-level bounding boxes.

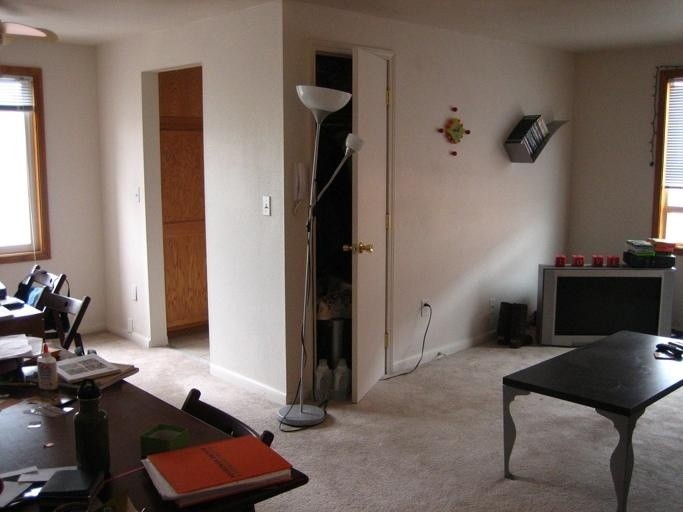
[277,83,364,427]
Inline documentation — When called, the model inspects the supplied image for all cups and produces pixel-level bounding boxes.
[555,256,619,267]
[73,378,109,472]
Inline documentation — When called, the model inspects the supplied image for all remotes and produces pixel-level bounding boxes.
[657,342,683,356]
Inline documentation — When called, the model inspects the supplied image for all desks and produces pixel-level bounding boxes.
[0,341,309,511]
[0,294,45,341]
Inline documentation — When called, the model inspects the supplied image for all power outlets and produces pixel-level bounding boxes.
[421,299,431,317]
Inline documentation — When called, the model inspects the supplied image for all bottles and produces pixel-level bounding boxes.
[316,359,350,400]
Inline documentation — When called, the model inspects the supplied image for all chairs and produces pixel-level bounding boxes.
[12,265,92,356]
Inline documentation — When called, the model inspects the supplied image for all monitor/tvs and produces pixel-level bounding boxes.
[536,263,677,347]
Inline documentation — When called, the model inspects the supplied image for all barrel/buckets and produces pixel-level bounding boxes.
[314,360,332,400]
[333,360,352,397]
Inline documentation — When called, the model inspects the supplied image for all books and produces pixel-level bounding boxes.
[625,237,676,257]
[142,434,294,506]
[57,353,139,391]
[0,333,43,387]
[506,114,549,156]
[0,297,26,322]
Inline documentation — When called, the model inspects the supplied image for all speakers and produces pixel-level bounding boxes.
[494,302,533,347]
